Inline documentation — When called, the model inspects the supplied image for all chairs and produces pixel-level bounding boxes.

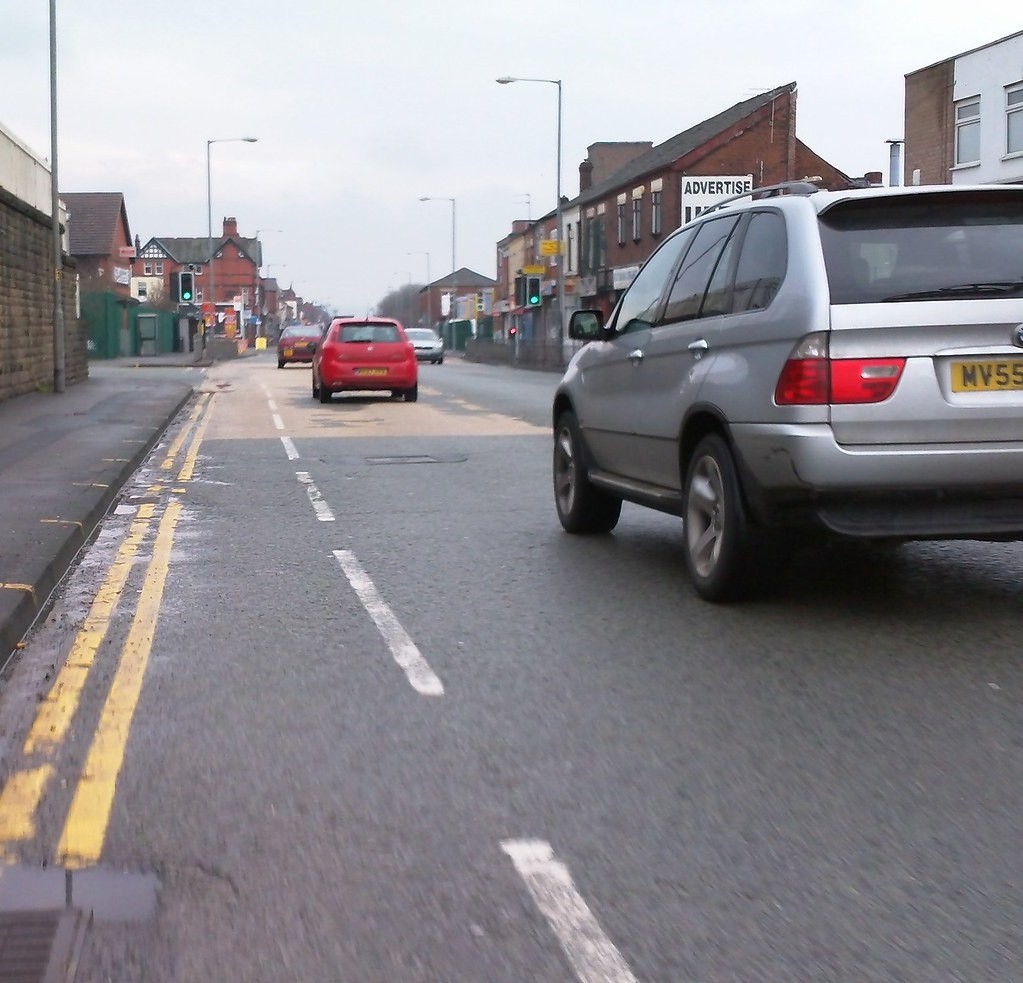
[825,252,869,303]
[342,329,362,341]
[892,241,959,292]
[374,329,390,342]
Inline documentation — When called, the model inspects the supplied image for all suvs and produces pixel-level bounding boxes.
[551,181,1023,606]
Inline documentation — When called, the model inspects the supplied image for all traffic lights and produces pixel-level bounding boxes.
[178,271,196,304]
[525,275,542,310]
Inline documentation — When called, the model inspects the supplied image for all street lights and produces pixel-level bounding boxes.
[495,75,572,374]
[206,137,257,360]
[419,195,456,355]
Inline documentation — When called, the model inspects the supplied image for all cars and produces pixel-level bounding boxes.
[404,327,443,364]
[307,314,417,405]
[276,324,327,370]
